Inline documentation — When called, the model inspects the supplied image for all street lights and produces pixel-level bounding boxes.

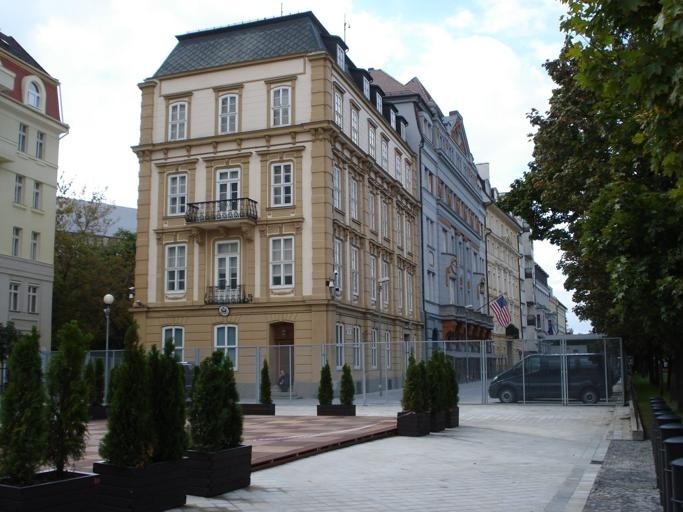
[464,305,472,384]
[378,277,389,396]
[102,294,114,406]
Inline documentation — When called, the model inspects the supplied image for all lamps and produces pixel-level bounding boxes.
[128,286,136,299]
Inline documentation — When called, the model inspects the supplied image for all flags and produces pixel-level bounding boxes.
[485,295,512,327]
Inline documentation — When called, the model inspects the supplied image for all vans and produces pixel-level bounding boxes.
[488,353,612,404]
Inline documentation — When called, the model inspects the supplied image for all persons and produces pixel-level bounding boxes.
[277,369,290,392]
